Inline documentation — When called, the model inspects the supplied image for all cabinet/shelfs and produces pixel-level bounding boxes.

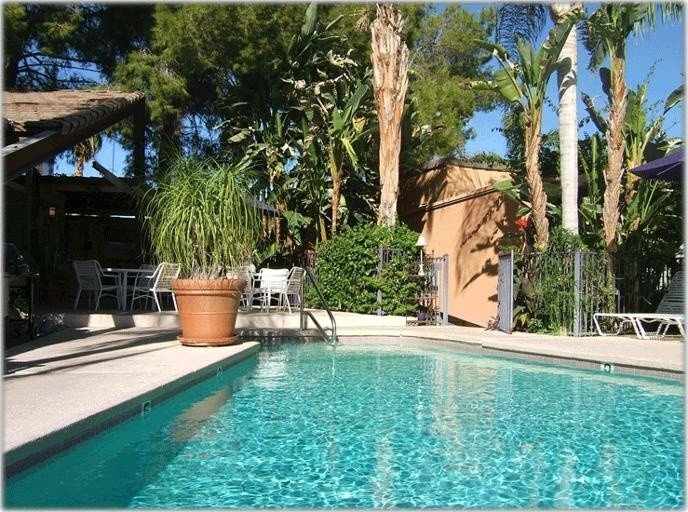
[404,257,437,325]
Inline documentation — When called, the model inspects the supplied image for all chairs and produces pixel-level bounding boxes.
[72,260,306,315]
[592,270,684,340]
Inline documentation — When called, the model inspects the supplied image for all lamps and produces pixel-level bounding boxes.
[415,234,427,277]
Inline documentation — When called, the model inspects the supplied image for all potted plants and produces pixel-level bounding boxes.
[137,132,277,347]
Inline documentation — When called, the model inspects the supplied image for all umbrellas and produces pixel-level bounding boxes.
[628,148,684,184]
